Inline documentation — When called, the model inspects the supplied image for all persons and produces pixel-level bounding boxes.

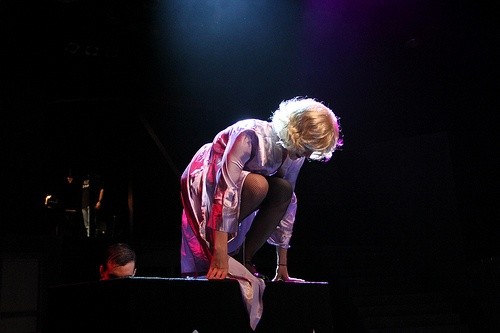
[74,177,137,281]
[180,95,343,331]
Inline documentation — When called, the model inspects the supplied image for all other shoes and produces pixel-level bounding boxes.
[239,258,267,280]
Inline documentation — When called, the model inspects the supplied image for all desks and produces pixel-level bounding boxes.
[36,276,330,333]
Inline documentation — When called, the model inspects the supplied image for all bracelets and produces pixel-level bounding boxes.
[278,264,287,266]
